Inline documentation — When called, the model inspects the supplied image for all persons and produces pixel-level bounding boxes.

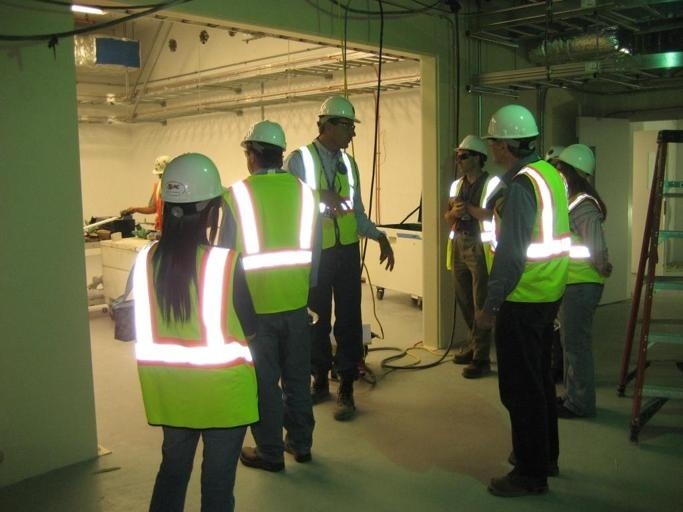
[215,118,323,474]
[542,142,567,387]
[440,130,510,381]
[283,93,396,425]
[471,103,573,497]
[119,154,172,235]
[131,151,261,511]
[555,139,613,420]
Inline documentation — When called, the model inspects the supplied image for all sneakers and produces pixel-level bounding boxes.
[549,361,598,420]
[282,439,313,464]
[486,464,550,498]
[452,350,474,365]
[239,444,287,472]
[461,358,495,380]
[505,449,562,477]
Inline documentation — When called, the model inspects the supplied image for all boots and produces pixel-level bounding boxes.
[332,372,357,421]
[306,370,332,406]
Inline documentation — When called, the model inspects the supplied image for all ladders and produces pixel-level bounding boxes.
[616,130,683,442]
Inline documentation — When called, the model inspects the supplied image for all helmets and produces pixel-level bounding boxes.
[315,96,361,124]
[453,133,488,159]
[480,102,542,141]
[153,155,175,176]
[545,143,598,178]
[240,120,287,150]
[159,152,225,203]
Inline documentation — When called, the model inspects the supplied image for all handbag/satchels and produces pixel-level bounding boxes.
[110,300,138,342]
